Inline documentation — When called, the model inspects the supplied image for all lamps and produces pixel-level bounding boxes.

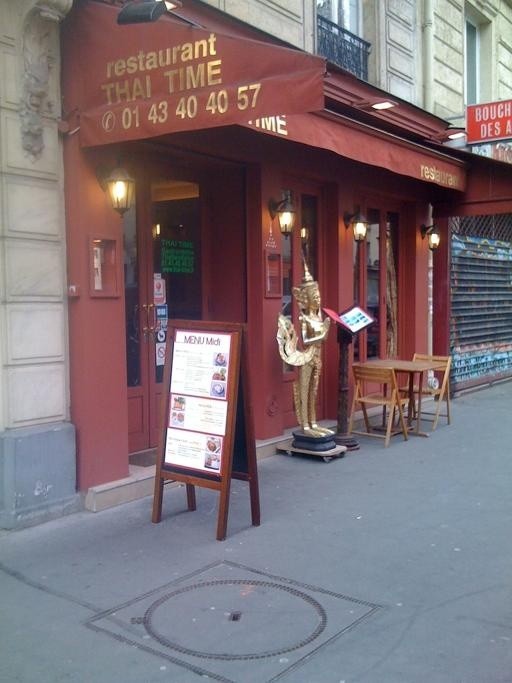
[269,190,297,239]
[95,149,137,218]
[357,96,398,111]
[344,203,368,242]
[116,0,167,25]
[430,127,468,144]
[420,218,441,251]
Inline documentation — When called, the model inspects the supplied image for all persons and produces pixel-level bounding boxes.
[277,285,334,437]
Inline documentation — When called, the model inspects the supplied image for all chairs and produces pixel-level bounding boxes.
[347,353,453,448]
